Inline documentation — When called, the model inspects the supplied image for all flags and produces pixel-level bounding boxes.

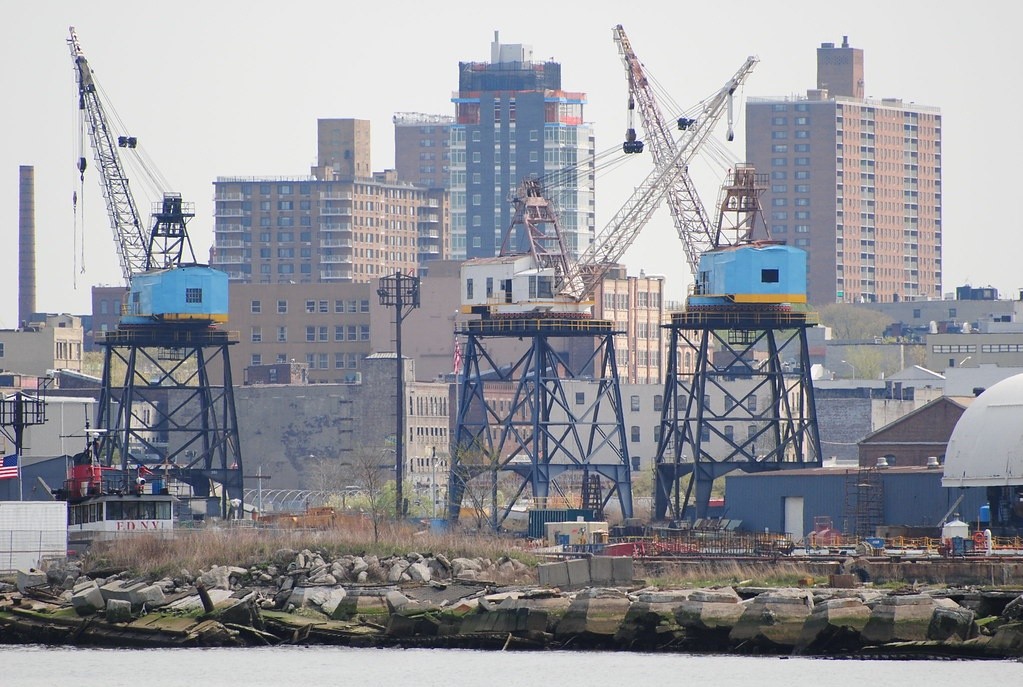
[0,453,18,480]
[454,324,462,375]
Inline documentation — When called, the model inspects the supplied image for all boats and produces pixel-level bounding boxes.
[50,402,182,557]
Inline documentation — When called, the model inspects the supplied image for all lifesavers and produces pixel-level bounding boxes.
[974,533,985,543]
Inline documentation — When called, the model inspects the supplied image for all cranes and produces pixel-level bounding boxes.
[66,24,246,523]
[611,24,828,532]
[444,56,763,531]
